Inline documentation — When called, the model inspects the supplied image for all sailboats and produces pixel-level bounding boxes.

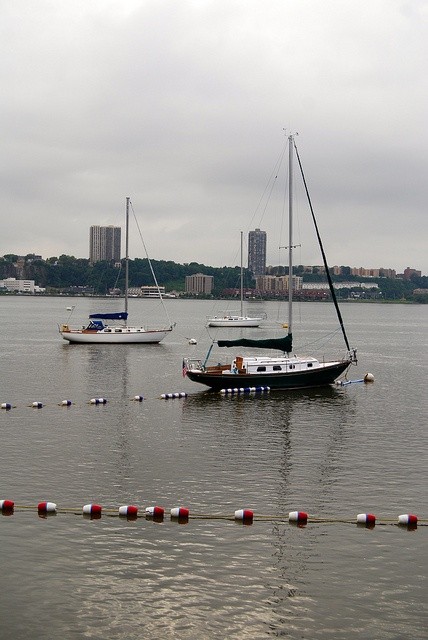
[54,195,176,344]
[181,127,357,390]
[204,229,262,328]
